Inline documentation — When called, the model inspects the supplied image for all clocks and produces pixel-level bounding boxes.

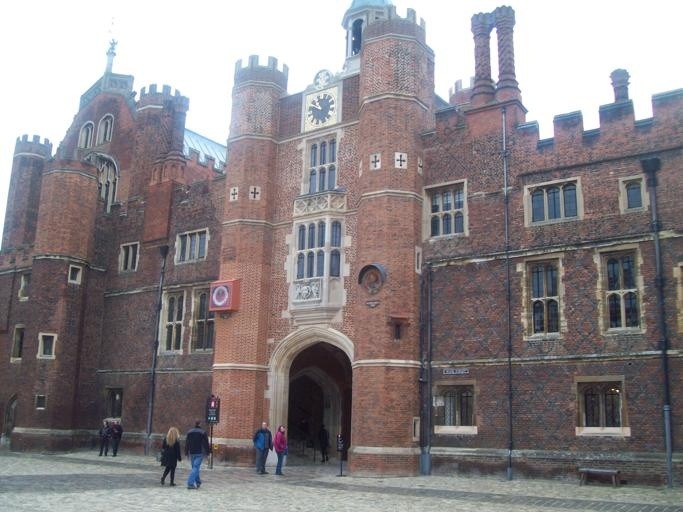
[305,90,336,128]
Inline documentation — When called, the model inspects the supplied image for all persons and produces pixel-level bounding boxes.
[251,420,288,474]
[318,423,330,463]
[160,419,211,488]
[98,420,123,456]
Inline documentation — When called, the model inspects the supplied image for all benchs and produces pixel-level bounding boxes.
[577,467,621,488]
